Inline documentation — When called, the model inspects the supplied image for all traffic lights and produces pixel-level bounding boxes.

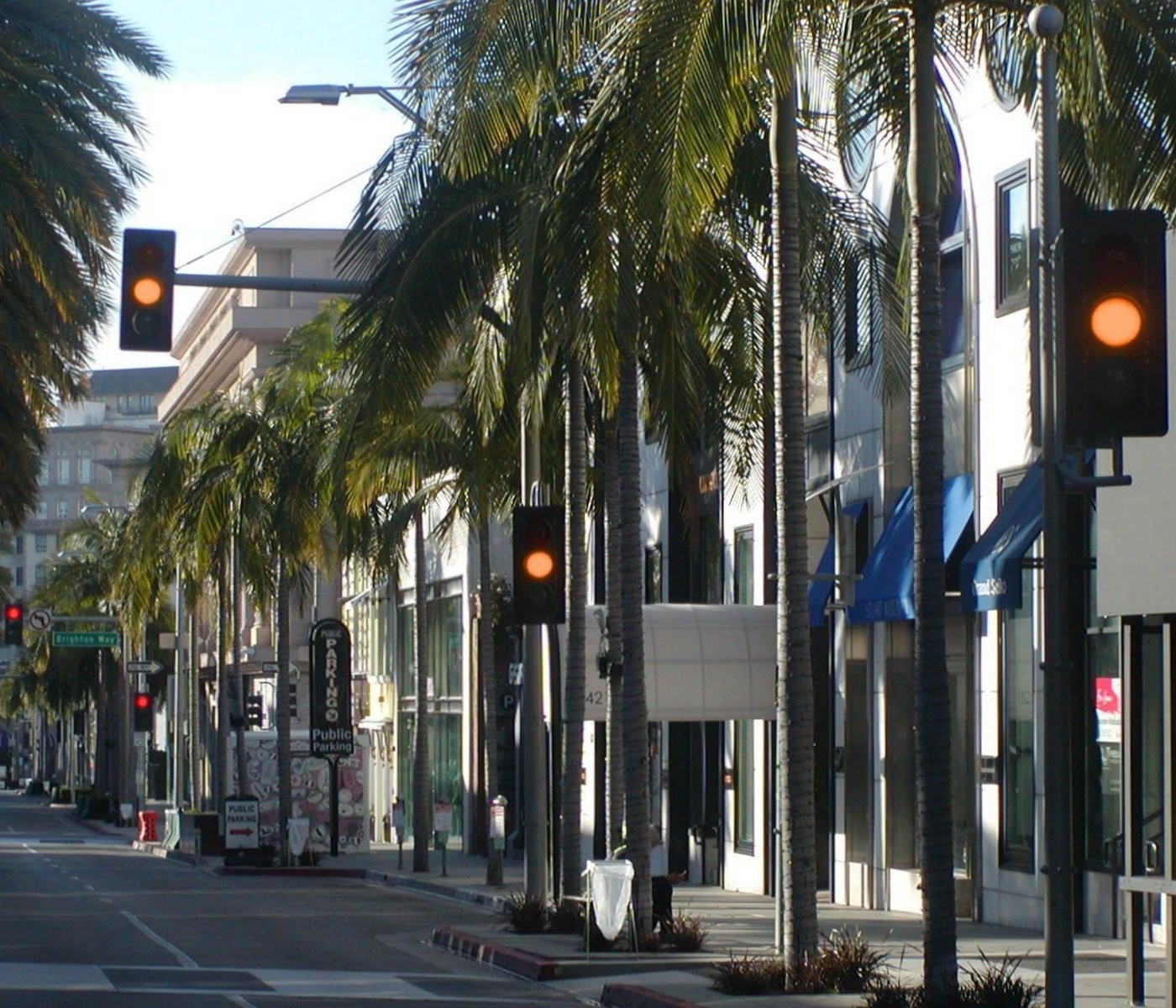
[125,659,166,675]
[118,228,177,352]
[511,505,567,625]
[4,604,24,646]
[1060,207,1170,439]
[132,691,153,733]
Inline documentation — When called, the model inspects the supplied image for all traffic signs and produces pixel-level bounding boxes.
[225,795,260,849]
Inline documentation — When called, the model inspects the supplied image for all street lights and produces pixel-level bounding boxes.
[81,503,184,812]
[276,83,549,922]
